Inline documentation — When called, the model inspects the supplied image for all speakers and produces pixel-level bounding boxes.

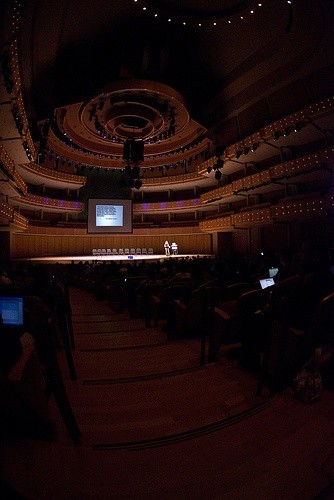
[124,141,144,160]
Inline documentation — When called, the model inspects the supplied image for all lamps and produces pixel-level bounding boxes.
[294,122,301,132]
[250,143,257,152]
[242,147,249,156]
[235,151,240,159]
[212,164,217,171]
[273,131,280,141]
[206,167,212,174]
[282,126,291,137]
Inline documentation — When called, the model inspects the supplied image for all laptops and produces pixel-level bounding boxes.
[269,268,279,278]
[259,277,275,290]
[0,296,25,327]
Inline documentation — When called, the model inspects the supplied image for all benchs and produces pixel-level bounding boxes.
[1,259,334,447]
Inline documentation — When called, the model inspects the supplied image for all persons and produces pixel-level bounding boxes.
[164,241,171,255]
[2,256,334,437]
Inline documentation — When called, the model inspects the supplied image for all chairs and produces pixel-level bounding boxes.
[92,248,153,256]
